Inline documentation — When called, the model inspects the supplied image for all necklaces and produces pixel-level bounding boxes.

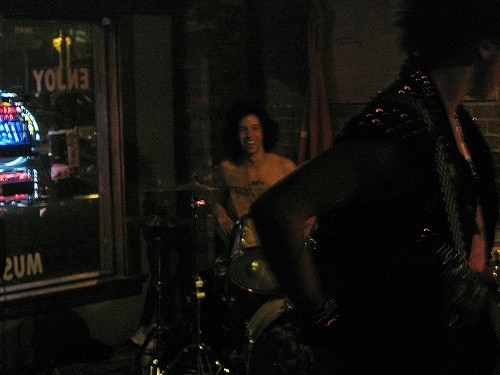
[448,111,478,177]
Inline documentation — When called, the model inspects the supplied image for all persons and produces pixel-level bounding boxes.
[209,104,316,247]
[250,0,500,375]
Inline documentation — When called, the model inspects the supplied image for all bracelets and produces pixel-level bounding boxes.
[304,222,315,230]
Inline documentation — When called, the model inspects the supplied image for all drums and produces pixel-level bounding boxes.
[238,295,315,375]
[225,246,282,299]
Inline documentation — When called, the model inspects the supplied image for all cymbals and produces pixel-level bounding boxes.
[144,178,225,195]
[126,214,175,231]
